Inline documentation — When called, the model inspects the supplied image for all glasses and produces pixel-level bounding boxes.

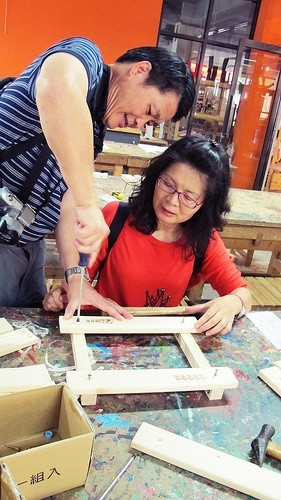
[156,176,205,209]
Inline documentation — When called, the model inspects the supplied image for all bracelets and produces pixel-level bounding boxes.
[230,291,248,320]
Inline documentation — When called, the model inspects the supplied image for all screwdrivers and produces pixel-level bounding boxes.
[104,186,125,199]
[76,251,89,322]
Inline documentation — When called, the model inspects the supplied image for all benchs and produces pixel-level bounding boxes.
[199,277,281,311]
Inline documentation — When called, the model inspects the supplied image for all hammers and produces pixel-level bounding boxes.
[251,423,281,475]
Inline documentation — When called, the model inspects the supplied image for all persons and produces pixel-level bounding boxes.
[43,135,253,338]
[0,37,195,308]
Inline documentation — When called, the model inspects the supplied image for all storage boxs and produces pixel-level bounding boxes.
[0,382,95,500]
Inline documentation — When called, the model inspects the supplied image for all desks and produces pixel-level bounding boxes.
[0,307,281,500]
[94,140,238,176]
[98,175,281,277]
[104,127,141,145]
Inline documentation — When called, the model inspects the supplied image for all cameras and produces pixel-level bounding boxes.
[0,186,36,244]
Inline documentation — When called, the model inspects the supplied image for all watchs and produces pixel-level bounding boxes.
[65,265,90,285]
